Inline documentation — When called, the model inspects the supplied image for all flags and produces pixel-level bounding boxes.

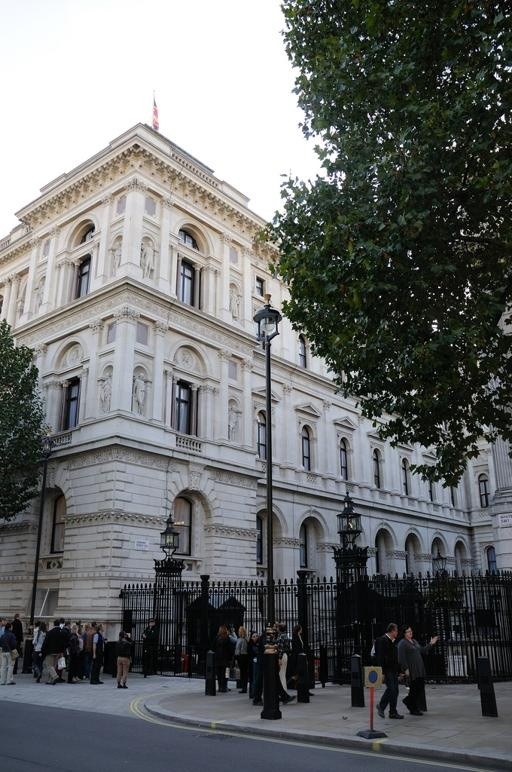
[153,96,158,130]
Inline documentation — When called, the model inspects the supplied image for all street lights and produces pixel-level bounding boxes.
[21,431,54,676]
[431,546,452,640]
[330,491,370,659]
[252,292,282,723]
[152,513,185,655]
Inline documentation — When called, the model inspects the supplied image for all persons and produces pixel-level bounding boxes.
[375,623,404,719]
[228,404,241,442]
[230,288,240,322]
[141,241,154,279]
[141,618,161,677]
[397,625,439,716]
[133,373,145,415]
[100,373,111,413]
[112,241,121,269]
[117,631,135,688]
[0,613,106,686]
[213,623,314,706]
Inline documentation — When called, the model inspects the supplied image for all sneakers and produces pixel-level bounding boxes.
[283,695,295,703]
[376,704,385,718]
[389,713,404,719]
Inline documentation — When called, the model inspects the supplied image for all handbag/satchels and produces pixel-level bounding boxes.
[12,649,20,660]
[225,667,231,679]
[58,657,66,670]
[232,663,241,680]
